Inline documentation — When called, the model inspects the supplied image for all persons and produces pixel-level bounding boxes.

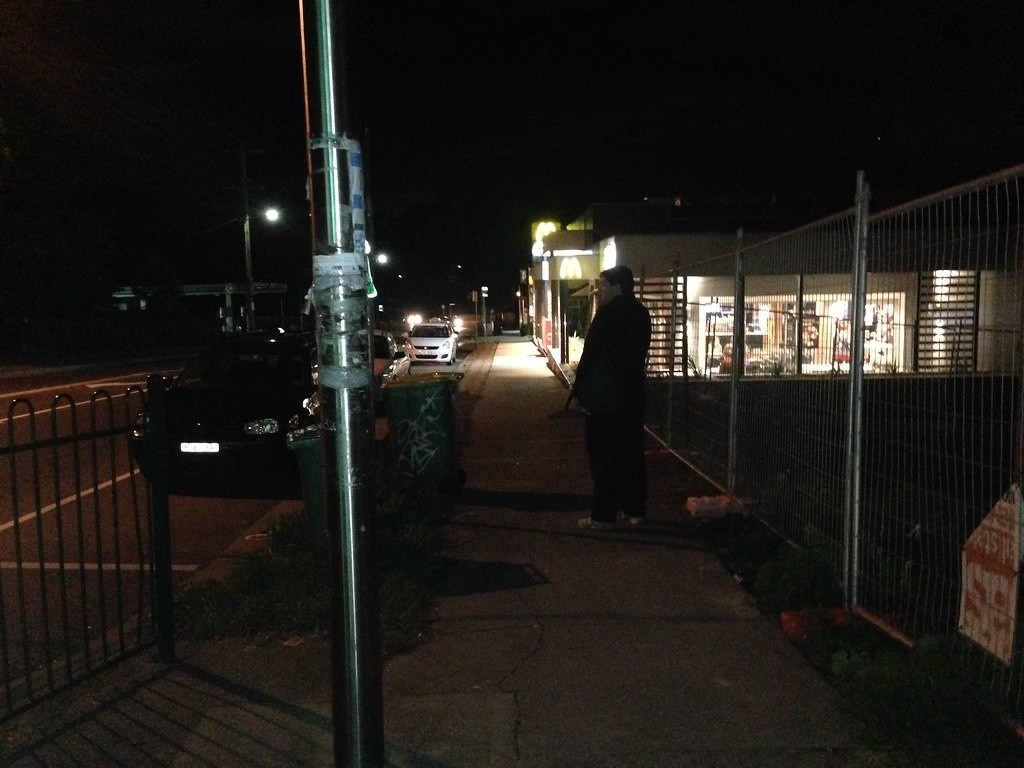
[564,267,652,530]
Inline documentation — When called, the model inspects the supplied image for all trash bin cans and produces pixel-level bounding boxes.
[288,425,331,554]
[381,371,468,492]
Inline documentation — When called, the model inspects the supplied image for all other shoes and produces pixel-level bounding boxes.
[577,516,616,530]
[620,510,645,525]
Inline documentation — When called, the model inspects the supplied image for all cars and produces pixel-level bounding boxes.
[129,332,318,489]
[401,324,458,365]
[349,328,413,386]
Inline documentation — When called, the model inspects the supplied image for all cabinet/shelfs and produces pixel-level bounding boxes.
[698,296,768,375]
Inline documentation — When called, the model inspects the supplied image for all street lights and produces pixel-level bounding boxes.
[481,286,488,338]
[450,303,455,316]
[244,210,278,332]
